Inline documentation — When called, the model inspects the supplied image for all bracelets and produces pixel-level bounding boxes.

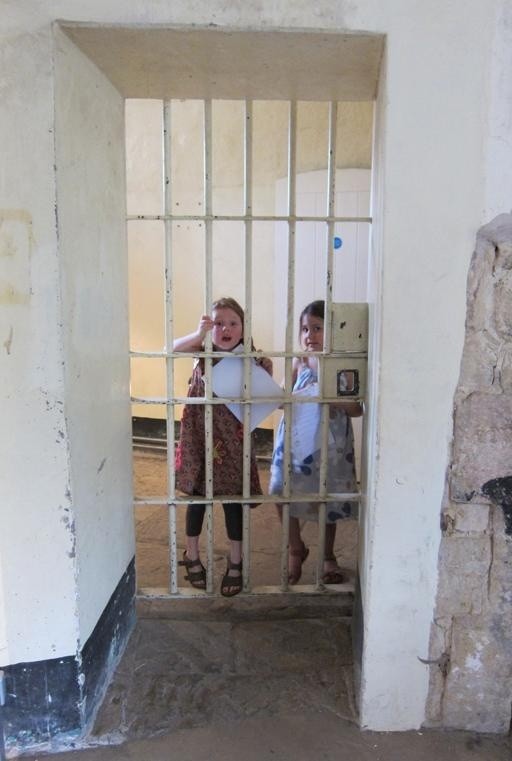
[194,328,210,344]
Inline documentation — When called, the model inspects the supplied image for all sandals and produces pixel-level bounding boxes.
[287,541,309,585]
[178,550,207,588]
[220,554,243,597]
[323,554,342,583]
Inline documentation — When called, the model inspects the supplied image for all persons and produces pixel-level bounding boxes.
[163,300,274,601]
[264,302,363,591]
